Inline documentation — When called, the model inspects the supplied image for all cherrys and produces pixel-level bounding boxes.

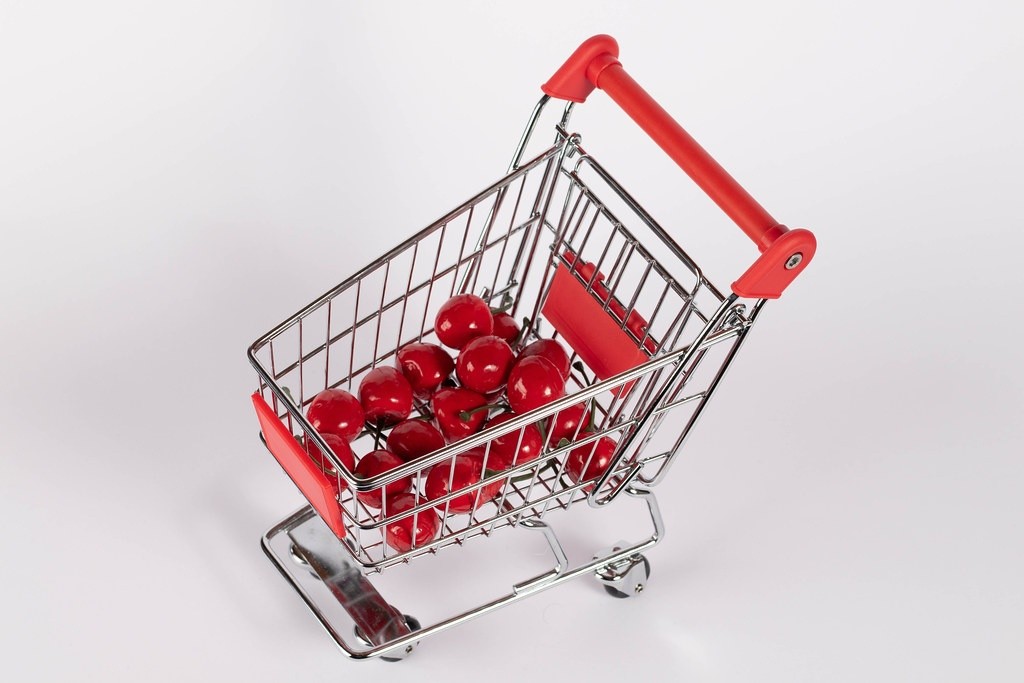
[294,295,620,554]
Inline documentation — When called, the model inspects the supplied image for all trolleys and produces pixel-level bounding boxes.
[246,35,817,667]
